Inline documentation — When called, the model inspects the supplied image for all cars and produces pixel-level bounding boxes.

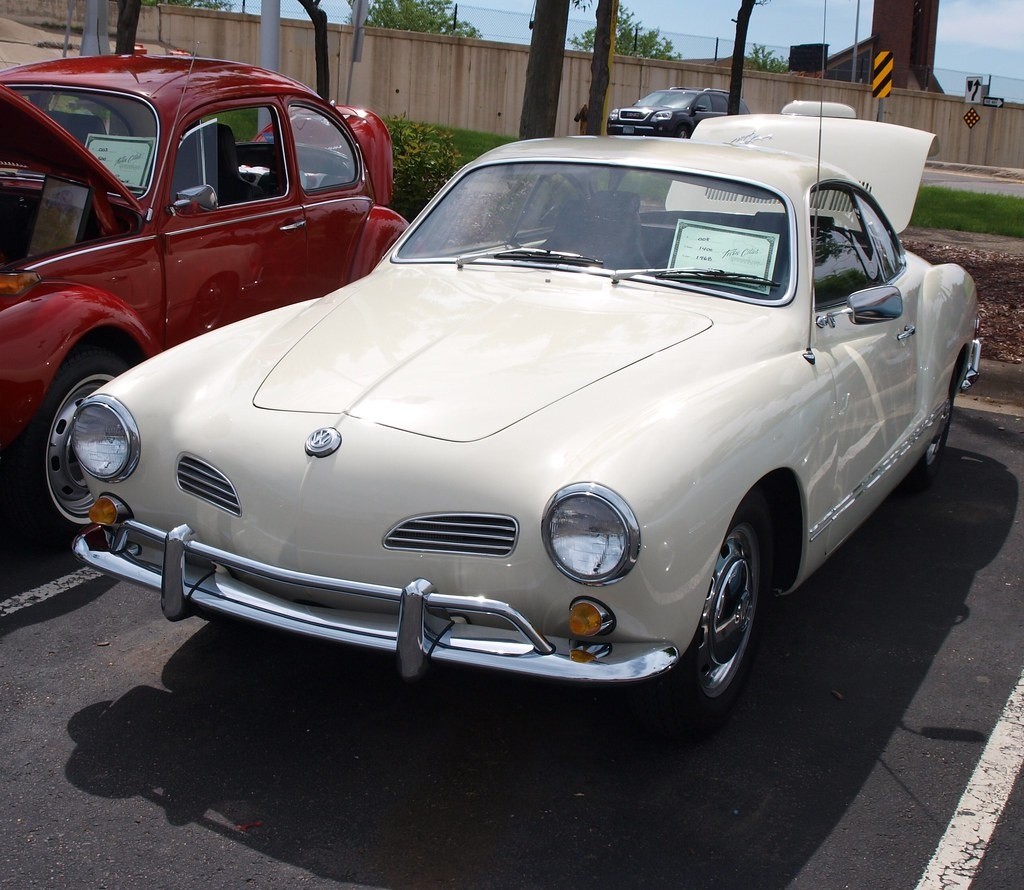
[0,45,409,557]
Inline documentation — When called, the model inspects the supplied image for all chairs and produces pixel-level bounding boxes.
[44,110,107,149]
[173,122,264,207]
[744,211,840,305]
[561,190,652,269]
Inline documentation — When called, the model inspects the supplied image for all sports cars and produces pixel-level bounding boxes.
[69,96,984,741]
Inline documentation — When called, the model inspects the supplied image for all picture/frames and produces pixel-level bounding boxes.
[22,173,95,262]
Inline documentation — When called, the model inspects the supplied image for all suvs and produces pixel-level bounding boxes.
[606,86,750,139]
